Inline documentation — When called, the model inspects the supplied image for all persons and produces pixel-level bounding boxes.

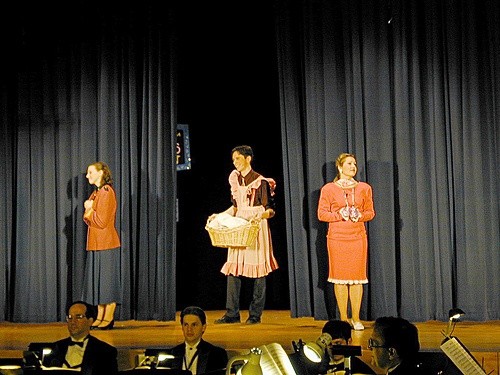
[164,307,228,375]
[208,146,275,325]
[81,162,122,331]
[291,316,433,375]
[42,301,118,372]
[318,153,376,331]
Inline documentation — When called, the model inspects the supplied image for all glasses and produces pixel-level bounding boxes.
[368,338,391,348]
[65,313,86,322]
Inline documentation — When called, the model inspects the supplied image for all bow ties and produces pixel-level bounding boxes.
[67,340,84,347]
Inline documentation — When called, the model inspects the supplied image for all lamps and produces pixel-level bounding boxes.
[145,348,175,368]
[303,333,333,363]
[448,306,465,337]
[29,342,54,364]
[225,347,263,375]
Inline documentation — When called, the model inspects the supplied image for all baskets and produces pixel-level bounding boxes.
[205,215,264,248]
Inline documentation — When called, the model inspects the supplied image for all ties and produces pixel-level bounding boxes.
[241,178,245,187]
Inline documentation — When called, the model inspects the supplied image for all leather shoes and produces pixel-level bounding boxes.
[94,319,115,330]
[245,315,262,324]
[90,321,102,330]
[350,318,365,331]
[220,312,241,323]
